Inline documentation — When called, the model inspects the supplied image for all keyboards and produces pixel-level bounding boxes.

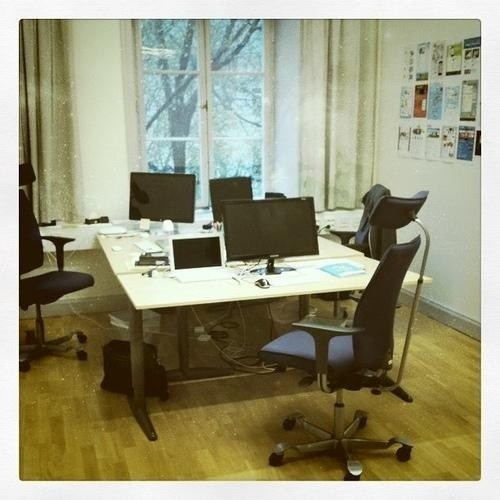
[134,240,162,252]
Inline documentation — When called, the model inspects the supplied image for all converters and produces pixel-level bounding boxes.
[194,326,205,333]
[198,334,211,342]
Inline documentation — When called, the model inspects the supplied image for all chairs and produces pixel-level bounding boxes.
[261,190,430,481]
[312,184,398,319]
[19,163,95,373]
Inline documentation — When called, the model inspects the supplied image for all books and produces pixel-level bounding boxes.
[320,261,366,278]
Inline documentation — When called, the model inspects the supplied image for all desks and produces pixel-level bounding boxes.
[37,208,364,254]
[96,231,432,441]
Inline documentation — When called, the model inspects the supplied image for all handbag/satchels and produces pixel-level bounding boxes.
[100,340,168,402]
[355,183,390,245]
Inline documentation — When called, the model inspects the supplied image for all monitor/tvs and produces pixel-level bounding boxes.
[209,177,252,223]
[127,171,195,223]
[219,196,319,275]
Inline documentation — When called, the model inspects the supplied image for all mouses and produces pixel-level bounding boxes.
[255,279,270,289]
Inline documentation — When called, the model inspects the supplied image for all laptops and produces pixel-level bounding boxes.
[169,235,233,283]
